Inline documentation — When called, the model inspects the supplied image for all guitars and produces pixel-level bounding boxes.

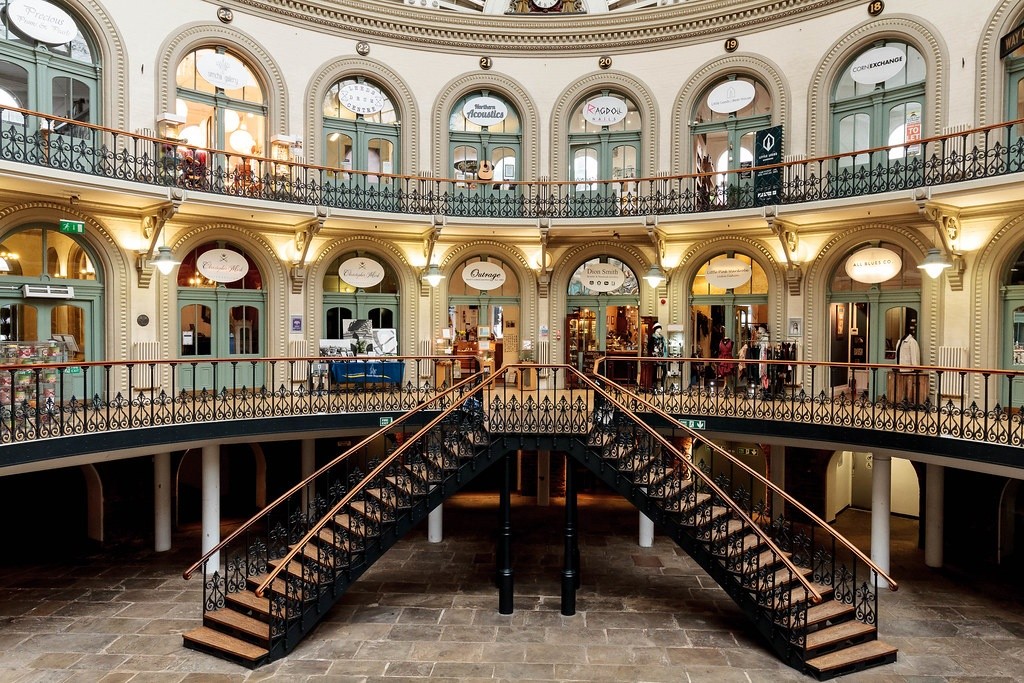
[478,147,493,180]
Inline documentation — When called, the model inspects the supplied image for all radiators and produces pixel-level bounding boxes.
[937,346,969,397]
[419,339,431,378]
[540,175,549,212]
[290,340,308,382]
[133,342,160,390]
[785,154,804,197]
[295,156,305,199]
[657,171,668,208]
[942,123,972,174]
[136,127,156,177]
[539,341,549,377]
[419,170,433,209]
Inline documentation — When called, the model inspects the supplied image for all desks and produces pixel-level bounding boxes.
[888,371,929,409]
[608,349,638,382]
[328,362,404,393]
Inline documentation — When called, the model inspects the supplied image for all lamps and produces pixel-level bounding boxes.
[916,226,953,279]
[423,250,446,289]
[147,225,181,275]
[642,244,667,289]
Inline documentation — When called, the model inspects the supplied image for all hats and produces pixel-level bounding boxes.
[652,321,662,333]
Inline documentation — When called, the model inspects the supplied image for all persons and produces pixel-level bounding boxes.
[755,324,769,348]
[791,322,800,335]
[895,332,920,373]
[647,323,667,395]
[717,337,735,377]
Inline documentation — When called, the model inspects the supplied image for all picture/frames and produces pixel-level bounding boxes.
[788,317,802,337]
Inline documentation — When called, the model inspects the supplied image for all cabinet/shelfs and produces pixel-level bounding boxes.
[567,314,601,352]
